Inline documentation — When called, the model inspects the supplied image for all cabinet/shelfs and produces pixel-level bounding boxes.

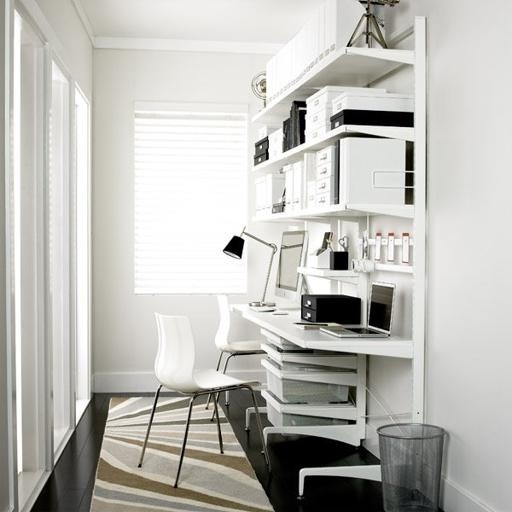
[227,14,427,512]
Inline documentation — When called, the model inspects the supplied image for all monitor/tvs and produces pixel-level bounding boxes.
[273,230,309,310]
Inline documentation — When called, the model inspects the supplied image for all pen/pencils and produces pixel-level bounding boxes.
[272,313,288,315]
[293,322,328,326]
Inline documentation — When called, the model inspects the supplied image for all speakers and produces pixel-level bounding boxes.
[330,251,348,269]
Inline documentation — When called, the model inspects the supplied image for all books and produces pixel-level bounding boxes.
[259,326,312,352]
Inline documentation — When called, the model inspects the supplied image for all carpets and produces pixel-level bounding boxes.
[90,396,275,512]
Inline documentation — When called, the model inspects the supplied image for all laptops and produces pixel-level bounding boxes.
[322,282,396,338]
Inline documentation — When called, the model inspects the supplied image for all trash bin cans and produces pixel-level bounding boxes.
[376,423,445,512]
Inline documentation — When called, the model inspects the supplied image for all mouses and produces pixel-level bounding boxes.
[272,311,288,315]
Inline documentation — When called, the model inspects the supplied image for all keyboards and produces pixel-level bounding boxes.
[252,307,274,312]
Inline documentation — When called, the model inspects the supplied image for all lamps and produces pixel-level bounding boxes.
[223,225,278,308]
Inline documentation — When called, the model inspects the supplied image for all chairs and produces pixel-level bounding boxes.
[138,312,272,488]
[205,294,267,422]
